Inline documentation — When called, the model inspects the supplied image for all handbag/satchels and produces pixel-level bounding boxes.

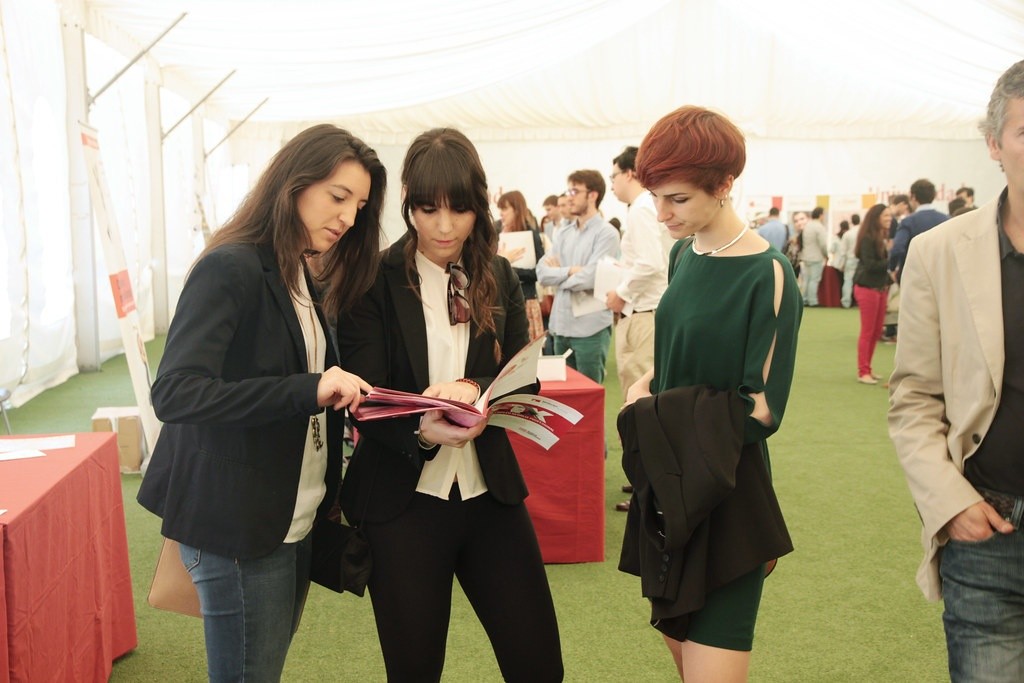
[310,518,371,597]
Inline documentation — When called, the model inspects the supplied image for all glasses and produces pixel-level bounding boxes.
[609,171,627,184]
[567,188,591,197]
[447,262,471,326]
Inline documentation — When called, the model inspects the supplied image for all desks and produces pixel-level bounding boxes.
[0,430,137,683]
[492,364,606,565]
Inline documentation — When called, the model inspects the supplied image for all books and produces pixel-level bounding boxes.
[353,332,584,451]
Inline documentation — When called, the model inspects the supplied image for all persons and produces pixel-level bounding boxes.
[792,212,811,256]
[336,127,564,682]
[886,59,1024,683]
[755,208,862,309]
[606,146,679,512]
[617,105,804,683]
[536,169,621,460]
[490,190,578,341]
[137,124,388,683]
[853,180,979,384]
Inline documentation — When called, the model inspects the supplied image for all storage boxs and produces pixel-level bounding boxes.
[90,407,145,474]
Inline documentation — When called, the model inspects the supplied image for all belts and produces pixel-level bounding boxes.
[974,486,1024,532]
[621,309,657,318]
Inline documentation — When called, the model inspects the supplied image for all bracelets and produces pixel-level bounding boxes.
[456,378,480,391]
[418,431,434,446]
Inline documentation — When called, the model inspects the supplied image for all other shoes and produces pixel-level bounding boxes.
[858,374,884,384]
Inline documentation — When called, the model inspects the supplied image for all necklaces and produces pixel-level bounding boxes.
[691,220,749,256]
[278,258,324,452]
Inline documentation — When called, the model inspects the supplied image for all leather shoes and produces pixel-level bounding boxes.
[616,500,630,511]
[622,486,634,493]
